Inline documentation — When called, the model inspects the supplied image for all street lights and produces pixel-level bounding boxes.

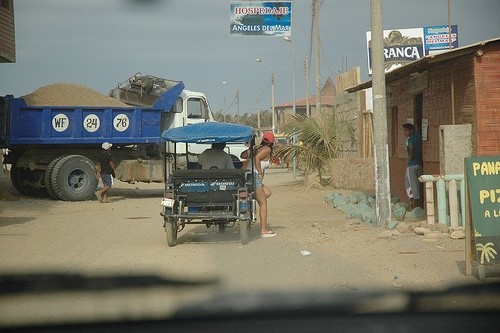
[255,58,276,135]
[284,37,297,178]
[222,81,241,125]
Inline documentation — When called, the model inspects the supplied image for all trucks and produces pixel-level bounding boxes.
[0,71,251,202]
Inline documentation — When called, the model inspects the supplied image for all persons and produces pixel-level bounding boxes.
[95,142,115,202]
[402,122,422,211]
[239,131,276,237]
[197,142,236,170]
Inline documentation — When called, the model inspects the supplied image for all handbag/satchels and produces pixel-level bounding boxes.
[110,167,115,178]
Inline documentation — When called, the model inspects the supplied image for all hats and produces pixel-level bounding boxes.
[262,132,275,144]
[101,142,113,150]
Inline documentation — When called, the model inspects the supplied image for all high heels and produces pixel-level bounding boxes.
[260,230,276,238]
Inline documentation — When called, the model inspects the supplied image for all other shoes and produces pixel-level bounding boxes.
[103,199,112,203]
[95,191,102,201]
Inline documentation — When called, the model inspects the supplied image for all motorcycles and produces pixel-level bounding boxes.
[160,121,257,247]
